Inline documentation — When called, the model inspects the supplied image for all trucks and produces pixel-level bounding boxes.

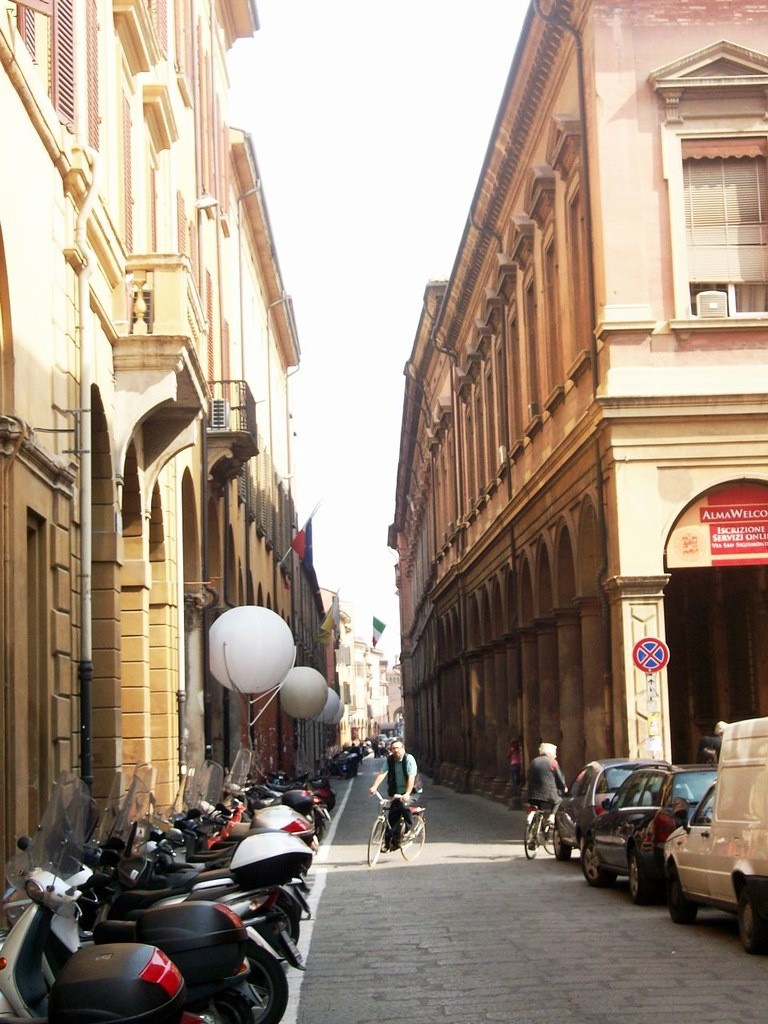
[663,717,768,956]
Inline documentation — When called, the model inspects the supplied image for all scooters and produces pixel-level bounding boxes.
[0,747,359,1024]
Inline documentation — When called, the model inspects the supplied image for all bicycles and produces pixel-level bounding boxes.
[366,791,427,868]
[524,788,565,860]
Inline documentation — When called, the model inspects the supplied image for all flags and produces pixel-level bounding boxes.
[372,617,385,647]
[291,518,313,570]
[312,594,339,645]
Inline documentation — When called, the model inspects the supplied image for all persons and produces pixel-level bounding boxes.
[507,733,520,796]
[367,741,422,852]
[351,736,390,766]
[526,743,568,850]
[696,722,727,762]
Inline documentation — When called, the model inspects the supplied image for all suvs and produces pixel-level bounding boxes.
[552,755,670,861]
[577,763,720,906]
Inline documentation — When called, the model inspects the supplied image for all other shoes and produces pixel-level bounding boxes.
[380,846,399,853]
[404,822,414,835]
[546,814,554,829]
[528,837,535,852]
